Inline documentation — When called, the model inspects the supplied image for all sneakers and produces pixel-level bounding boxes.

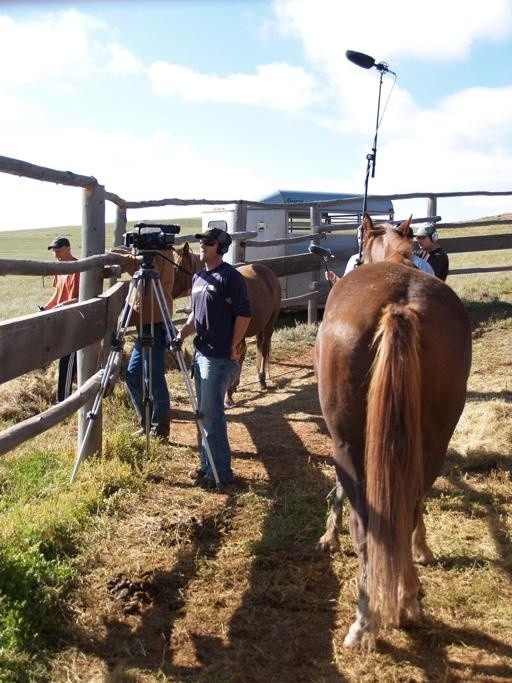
[146,431,170,443]
[131,427,149,437]
[189,466,204,479]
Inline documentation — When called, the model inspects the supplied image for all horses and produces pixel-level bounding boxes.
[310,212,475,653]
[169,240,282,409]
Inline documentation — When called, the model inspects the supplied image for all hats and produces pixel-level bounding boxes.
[195,227,231,253]
[413,223,436,238]
[47,236,70,249]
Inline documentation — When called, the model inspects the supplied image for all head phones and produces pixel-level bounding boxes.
[431,232,438,242]
[216,235,232,255]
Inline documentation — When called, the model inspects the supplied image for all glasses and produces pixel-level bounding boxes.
[198,235,216,247]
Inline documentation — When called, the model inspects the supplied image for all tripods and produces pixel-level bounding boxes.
[70,263,223,491]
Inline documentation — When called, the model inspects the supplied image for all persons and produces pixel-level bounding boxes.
[412,224,449,283]
[39,238,81,404]
[324,225,363,285]
[109,242,175,440]
[168,228,253,483]
